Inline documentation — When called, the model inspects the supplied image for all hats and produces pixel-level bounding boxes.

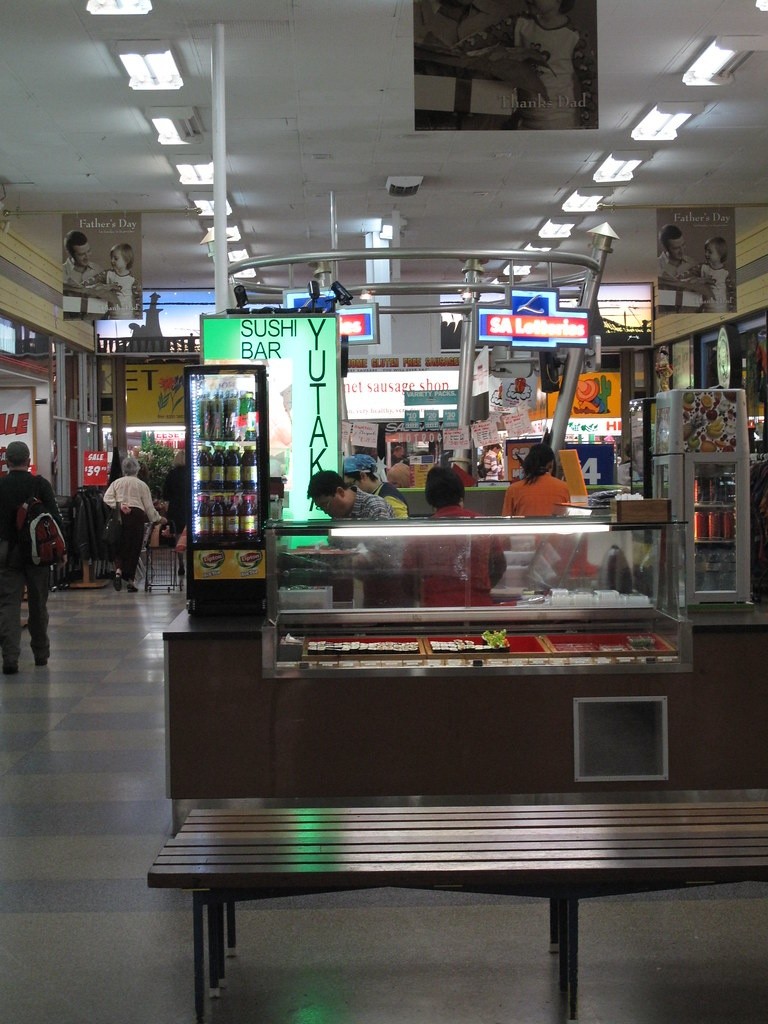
[343,455,376,473]
[6,441,30,461]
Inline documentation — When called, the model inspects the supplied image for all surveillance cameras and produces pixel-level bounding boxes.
[233,285,248,307]
[308,281,321,299]
[331,281,353,305]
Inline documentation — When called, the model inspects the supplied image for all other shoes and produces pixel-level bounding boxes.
[113,573,121,591]
[4,666,17,674]
[36,656,49,665]
[127,584,137,591]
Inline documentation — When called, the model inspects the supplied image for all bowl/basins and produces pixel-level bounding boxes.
[504,535,535,586]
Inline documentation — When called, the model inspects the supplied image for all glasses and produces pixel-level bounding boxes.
[317,493,336,512]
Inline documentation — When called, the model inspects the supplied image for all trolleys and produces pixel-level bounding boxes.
[142,518,184,593]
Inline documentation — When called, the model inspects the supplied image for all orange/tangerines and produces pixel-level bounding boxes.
[683,393,695,403]
[701,395,712,407]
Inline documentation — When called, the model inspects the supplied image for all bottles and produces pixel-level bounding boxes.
[694,548,735,592]
[196,392,256,538]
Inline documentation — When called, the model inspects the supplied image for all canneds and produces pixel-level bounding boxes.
[199,397,240,441]
[693,475,735,540]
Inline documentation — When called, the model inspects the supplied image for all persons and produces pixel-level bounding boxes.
[0,442,66,672]
[103,458,163,592]
[484,444,503,481]
[413,0,598,130]
[502,444,570,516]
[391,446,407,465]
[403,468,507,605]
[63,231,143,318]
[129,446,148,458]
[343,455,409,520]
[307,471,399,598]
[657,224,735,313]
[161,450,186,574]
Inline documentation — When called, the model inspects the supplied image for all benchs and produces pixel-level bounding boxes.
[148,801,768,1023]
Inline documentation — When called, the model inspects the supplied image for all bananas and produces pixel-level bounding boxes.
[706,418,725,437]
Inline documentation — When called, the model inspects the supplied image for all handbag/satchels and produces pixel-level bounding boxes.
[104,502,122,542]
[477,462,491,476]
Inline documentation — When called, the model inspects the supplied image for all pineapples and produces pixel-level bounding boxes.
[683,413,706,439]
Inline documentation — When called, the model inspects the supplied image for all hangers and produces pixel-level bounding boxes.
[76,487,95,495]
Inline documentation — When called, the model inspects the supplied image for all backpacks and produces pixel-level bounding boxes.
[9,474,65,568]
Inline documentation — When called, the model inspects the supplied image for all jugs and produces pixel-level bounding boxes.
[599,545,632,593]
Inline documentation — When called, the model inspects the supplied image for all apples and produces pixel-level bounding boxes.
[688,410,732,452]
